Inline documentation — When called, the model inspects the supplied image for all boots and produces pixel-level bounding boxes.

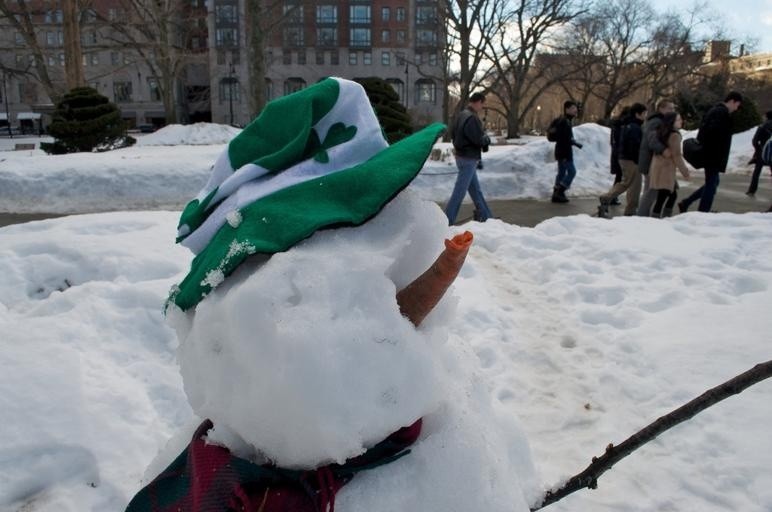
[551,184,570,203]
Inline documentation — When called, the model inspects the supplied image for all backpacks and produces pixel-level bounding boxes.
[761,136,772,164]
[547,114,562,142]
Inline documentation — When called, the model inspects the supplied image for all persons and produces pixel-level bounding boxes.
[598,101,648,218]
[550,100,585,205]
[638,97,675,216]
[745,110,771,196]
[647,111,690,220]
[609,106,631,206]
[443,92,492,225]
[677,89,741,214]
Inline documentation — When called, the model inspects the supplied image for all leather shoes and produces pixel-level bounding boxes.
[599,194,688,218]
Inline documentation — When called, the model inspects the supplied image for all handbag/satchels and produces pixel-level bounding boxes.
[682,138,709,169]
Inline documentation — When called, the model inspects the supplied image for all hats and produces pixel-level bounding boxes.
[161,76,449,317]
